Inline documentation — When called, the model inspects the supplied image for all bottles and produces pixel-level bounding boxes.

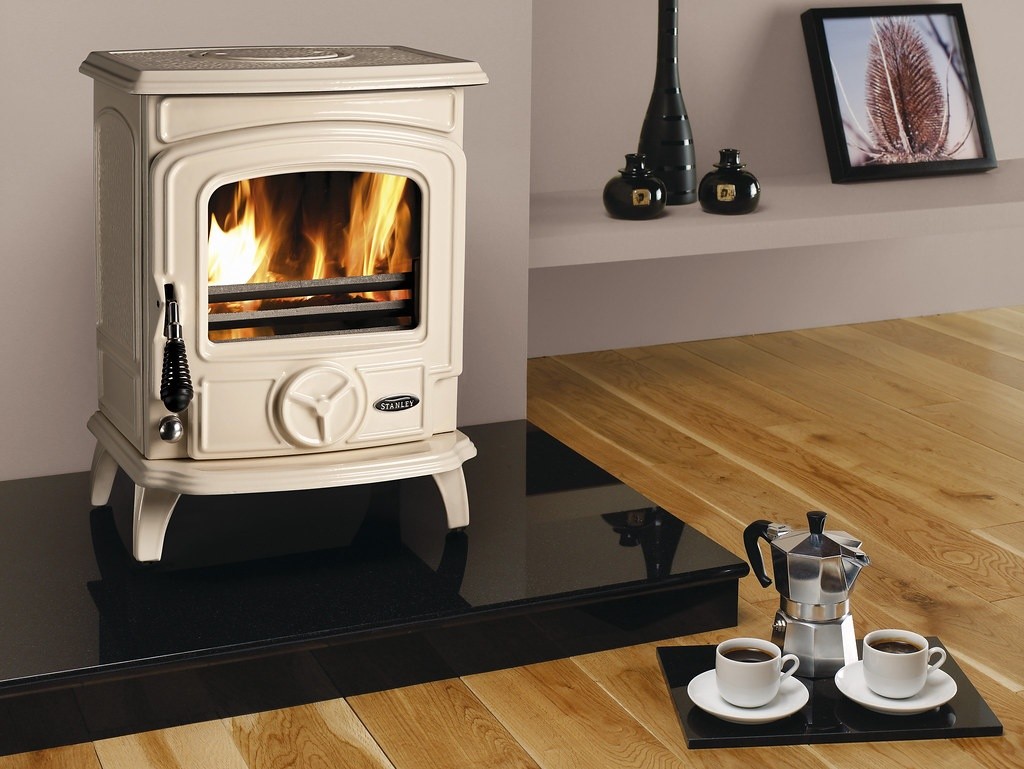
[698,149,761,215]
[603,153,667,220]
[638,0,697,204]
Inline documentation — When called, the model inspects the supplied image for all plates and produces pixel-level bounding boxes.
[835,660,958,716]
[687,668,809,725]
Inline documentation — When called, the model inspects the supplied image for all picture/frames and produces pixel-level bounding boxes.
[801,3,998,183]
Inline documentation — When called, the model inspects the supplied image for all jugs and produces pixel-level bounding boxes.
[743,511,869,677]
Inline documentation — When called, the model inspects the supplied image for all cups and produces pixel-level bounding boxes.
[715,637,800,708]
[863,628,947,698]
